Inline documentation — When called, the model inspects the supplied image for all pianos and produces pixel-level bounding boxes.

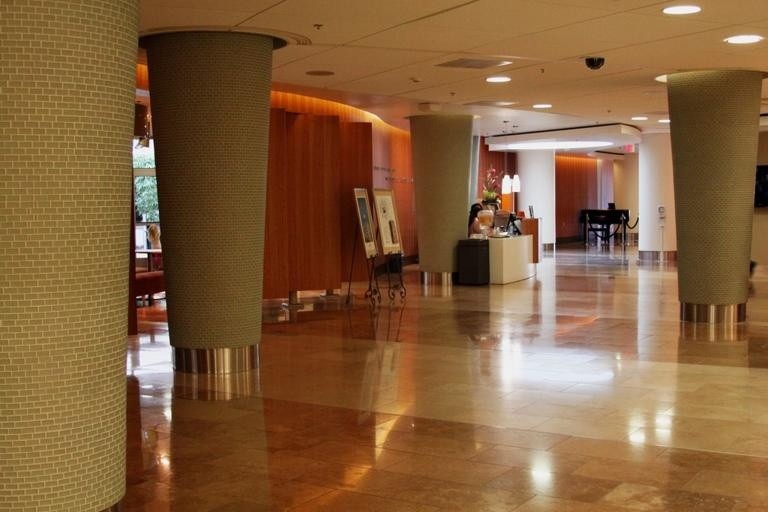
[580,208,630,248]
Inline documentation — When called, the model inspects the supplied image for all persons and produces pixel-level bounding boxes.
[468,203,484,239]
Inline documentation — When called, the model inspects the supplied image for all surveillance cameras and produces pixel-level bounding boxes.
[585,58,604,70]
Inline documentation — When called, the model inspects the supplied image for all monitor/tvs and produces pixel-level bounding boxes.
[608,203,615,209]
[755,165,768,207]
[506,212,516,231]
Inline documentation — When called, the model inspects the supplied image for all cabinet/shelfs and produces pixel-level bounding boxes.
[523,218,538,263]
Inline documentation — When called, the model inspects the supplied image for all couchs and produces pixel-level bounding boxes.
[135,249,167,305]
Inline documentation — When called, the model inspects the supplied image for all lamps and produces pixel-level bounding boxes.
[501,175,521,215]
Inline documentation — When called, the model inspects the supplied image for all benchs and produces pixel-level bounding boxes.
[588,228,609,243]
